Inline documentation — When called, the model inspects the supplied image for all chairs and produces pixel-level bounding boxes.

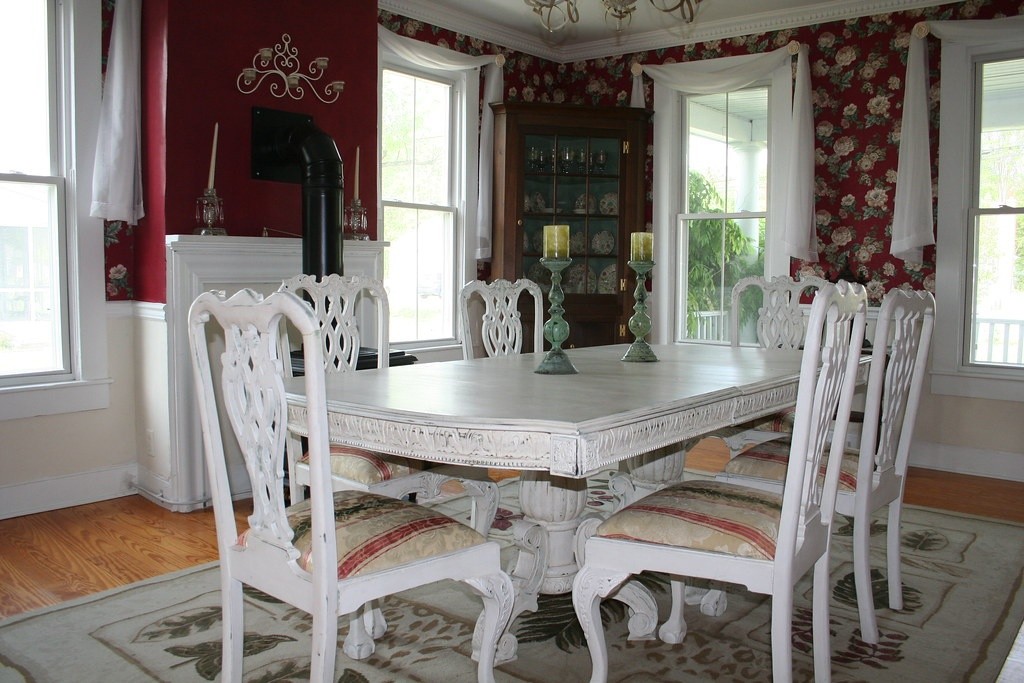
[697,288,939,646]
[275,272,501,643]
[683,278,832,469]
[572,278,869,683]
[188,288,517,683]
[457,278,544,362]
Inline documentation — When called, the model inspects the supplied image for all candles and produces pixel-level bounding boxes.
[542,224,570,258]
[354,143,360,203]
[630,232,654,262]
[208,121,219,192]
[243,46,346,94]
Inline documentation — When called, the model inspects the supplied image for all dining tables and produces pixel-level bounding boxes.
[282,342,875,669]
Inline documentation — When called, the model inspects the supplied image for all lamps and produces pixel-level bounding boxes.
[524,0,702,35]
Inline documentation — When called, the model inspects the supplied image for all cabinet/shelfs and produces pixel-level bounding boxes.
[488,100,648,354]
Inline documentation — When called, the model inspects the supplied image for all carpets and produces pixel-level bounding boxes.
[0,462,1024,683]
